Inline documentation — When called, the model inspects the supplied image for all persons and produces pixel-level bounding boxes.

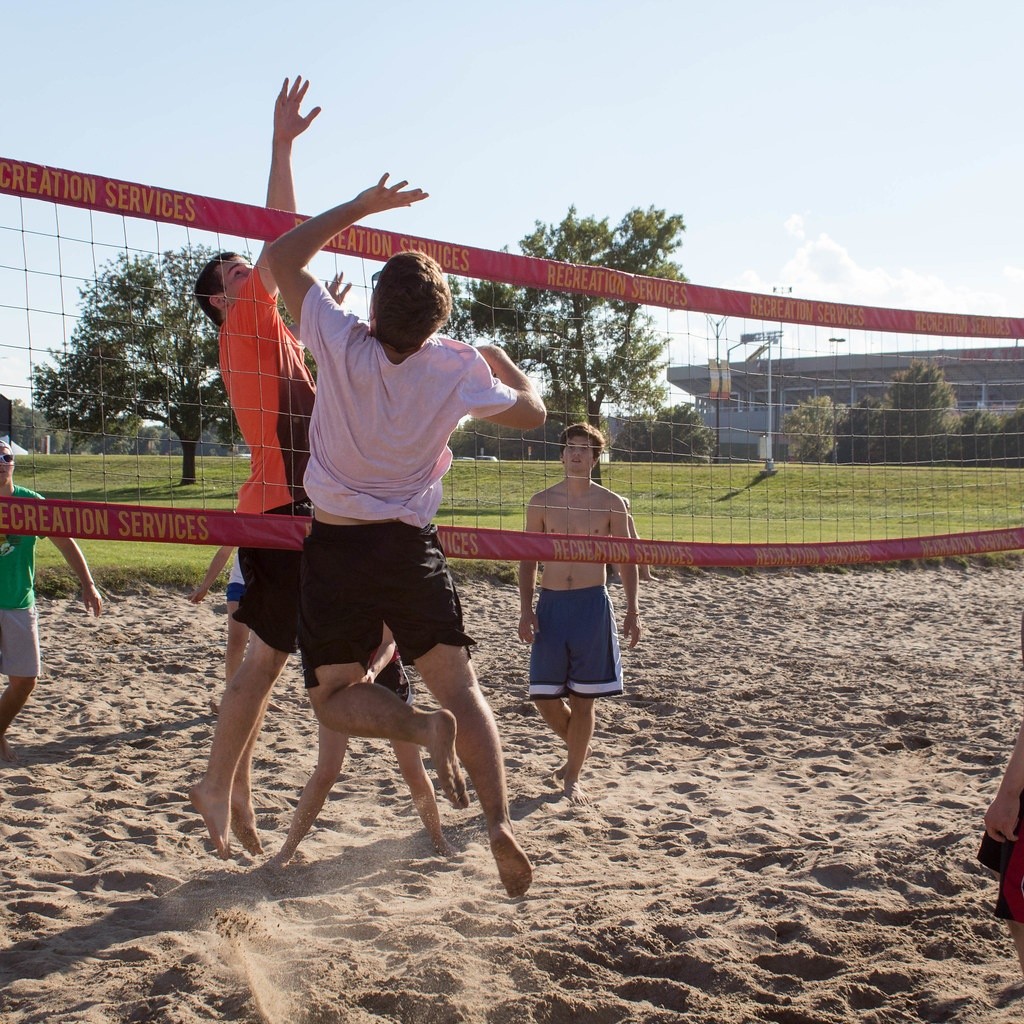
[0,440,102,761]
[281,623,459,865]
[267,173,547,897]
[190,76,352,861]
[610,497,663,582]
[977,610,1024,974]
[518,422,642,807]
[185,546,251,712]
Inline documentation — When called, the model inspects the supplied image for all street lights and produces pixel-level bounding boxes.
[741,334,775,472]
[828,337,846,463]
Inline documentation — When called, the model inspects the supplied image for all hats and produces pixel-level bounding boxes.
[0,440,13,455]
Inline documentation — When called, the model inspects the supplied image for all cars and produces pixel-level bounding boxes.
[456,455,498,460]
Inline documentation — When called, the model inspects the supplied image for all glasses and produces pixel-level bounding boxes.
[0,455,14,463]
[371,271,383,297]
